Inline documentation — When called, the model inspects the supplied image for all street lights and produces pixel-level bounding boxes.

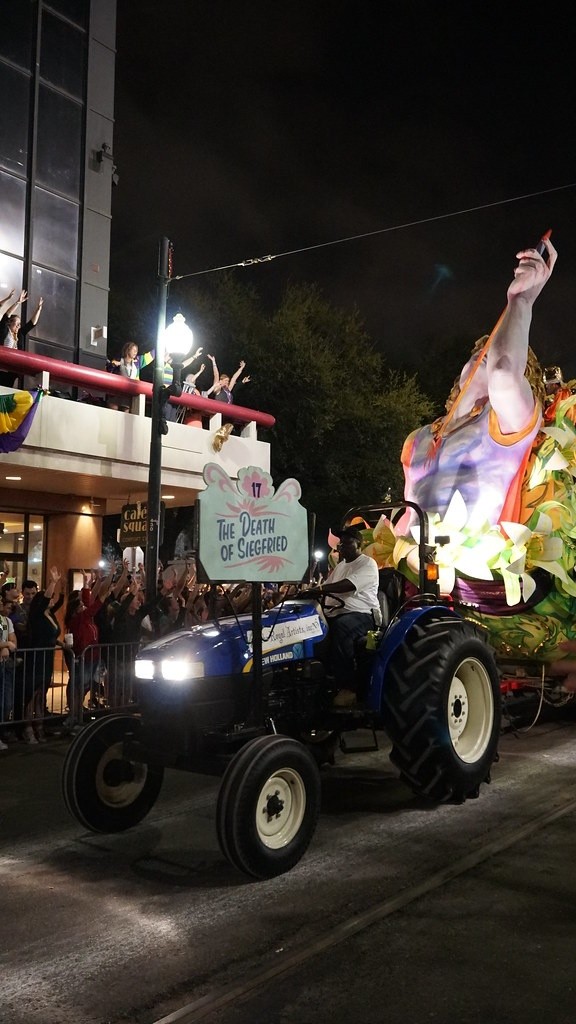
[146,305,196,640]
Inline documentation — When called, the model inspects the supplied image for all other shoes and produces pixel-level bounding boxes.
[35,727,47,743]
[23,731,38,745]
[0,740,8,750]
[334,689,357,706]
[3,736,18,742]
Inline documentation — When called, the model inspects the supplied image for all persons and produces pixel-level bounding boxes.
[111,342,251,435]
[400,227,559,580]
[0,288,44,388]
[0,525,380,751]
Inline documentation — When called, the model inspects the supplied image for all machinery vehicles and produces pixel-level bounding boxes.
[59,504,576,878]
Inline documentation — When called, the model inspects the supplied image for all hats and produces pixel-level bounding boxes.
[341,527,362,541]
